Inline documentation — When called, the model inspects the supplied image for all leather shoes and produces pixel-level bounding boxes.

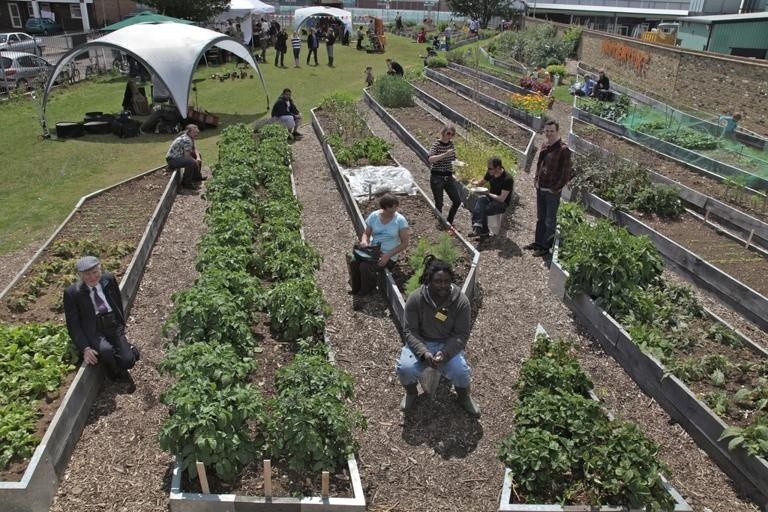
[467,227,481,236]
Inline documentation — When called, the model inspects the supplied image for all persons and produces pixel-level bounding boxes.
[62,254,141,385]
[356,20,383,51]
[165,124,207,190]
[395,12,403,29]
[274,29,288,66]
[464,157,515,255]
[522,119,577,259]
[416,16,479,67]
[530,65,610,100]
[322,28,335,66]
[223,18,280,62]
[395,256,483,417]
[365,67,374,86]
[306,28,319,65]
[428,121,462,234]
[270,88,303,140]
[290,32,302,67]
[385,59,403,77]
[347,194,410,297]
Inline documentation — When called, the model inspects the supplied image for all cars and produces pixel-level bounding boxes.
[0,33,47,58]
[24,16,63,37]
[0,51,70,93]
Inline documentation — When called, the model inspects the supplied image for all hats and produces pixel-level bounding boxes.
[75,256,100,274]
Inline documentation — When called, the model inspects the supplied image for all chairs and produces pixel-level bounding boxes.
[120,20,346,80]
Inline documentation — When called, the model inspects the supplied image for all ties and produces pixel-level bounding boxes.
[91,284,109,317]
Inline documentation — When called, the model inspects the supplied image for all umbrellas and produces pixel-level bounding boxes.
[93,8,197,32]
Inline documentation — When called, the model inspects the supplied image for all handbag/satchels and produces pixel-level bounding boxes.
[354,244,382,262]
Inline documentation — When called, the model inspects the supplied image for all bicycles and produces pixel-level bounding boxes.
[63,61,81,87]
[112,53,129,71]
[84,54,104,79]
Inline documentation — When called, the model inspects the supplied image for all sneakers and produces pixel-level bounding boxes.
[455,393,481,417]
[524,243,548,256]
[400,387,418,411]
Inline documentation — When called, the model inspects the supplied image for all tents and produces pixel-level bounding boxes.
[197,0,276,49]
[295,7,352,46]
[40,14,270,138]
